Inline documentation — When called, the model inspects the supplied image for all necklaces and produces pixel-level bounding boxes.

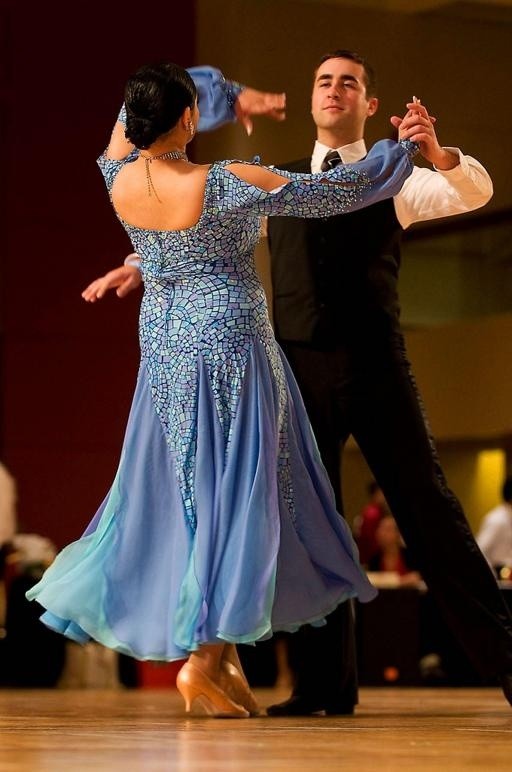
[137,152,189,204]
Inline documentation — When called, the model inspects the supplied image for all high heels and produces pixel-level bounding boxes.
[174,660,259,719]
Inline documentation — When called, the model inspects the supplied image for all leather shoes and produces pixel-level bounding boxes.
[264,686,361,719]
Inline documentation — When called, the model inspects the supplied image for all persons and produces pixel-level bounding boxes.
[25,64,434,716]
[80,50,511,717]
[349,476,421,577]
[472,478,509,581]
